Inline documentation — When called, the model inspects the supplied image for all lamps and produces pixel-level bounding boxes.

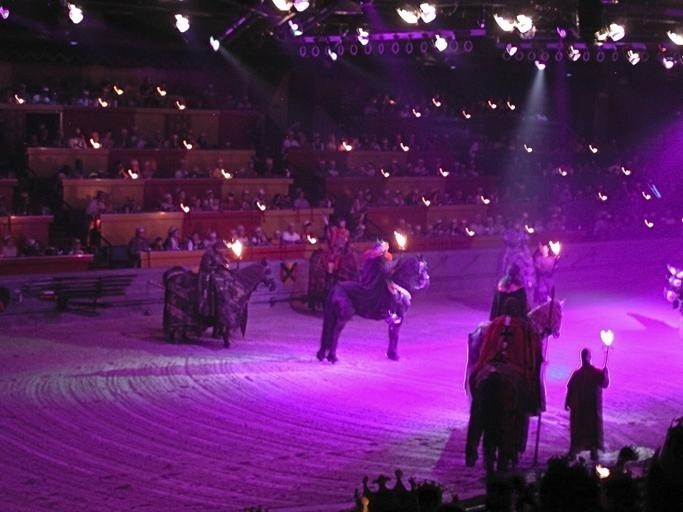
[271,0,683,70]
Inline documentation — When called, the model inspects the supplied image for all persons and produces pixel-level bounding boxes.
[490,263,526,321]
[565,348,609,461]
[475,298,539,388]
[360,241,400,324]
[198,242,229,292]
[397,75,682,239]
[2,74,404,264]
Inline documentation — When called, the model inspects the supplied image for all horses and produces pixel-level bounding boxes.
[463,295,567,396]
[478,372,528,474]
[162,256,276,348]
[316,253,429,364]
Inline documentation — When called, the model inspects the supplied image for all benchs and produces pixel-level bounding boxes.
[0,50,599,275]
[20,272,138,316]
